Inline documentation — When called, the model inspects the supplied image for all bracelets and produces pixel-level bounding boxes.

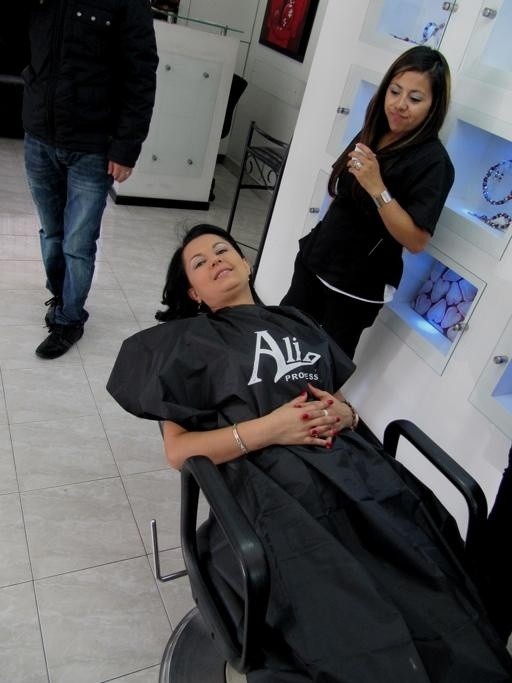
[232,422,248,454]
[374,189,393,209]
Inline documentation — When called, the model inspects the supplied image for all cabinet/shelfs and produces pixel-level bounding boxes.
[228,120,293,280]
[247,1,511,541]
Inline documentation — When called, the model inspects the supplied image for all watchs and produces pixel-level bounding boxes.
[343,398,359,431]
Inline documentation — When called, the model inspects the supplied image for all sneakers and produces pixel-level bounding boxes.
[35,296,90,360]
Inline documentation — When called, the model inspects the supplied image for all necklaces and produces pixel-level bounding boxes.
[462,160,512,231]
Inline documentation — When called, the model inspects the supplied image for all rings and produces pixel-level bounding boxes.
[324,409,328,416]
[353,161,362,170]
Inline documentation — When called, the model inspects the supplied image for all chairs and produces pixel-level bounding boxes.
[148,420,511,683]
[208,74,247,202]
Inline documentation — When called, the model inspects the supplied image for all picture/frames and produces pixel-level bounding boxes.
[257,1,320,63]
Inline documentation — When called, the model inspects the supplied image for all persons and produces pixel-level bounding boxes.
[23,0,158,361]
[157,222,512,682]
[278,46,456,358]
